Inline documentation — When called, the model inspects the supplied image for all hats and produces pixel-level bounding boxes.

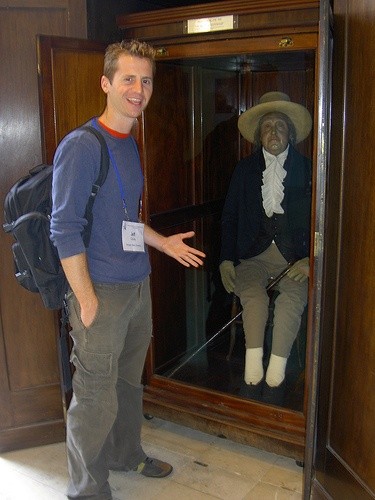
[237,90,313,148]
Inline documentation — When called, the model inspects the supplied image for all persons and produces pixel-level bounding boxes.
[219,93,314,408]
[50,40,207,500]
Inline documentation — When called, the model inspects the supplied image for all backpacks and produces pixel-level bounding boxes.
[2,125,111,309]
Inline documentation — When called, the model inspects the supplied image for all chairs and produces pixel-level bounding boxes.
[226,280,303,367]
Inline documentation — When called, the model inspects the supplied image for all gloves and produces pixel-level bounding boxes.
[287,257,311,283]
[219,259,239,294]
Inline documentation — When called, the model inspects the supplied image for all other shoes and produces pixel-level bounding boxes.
[238,371,264,402]
[262,371,287,406]
[130,457,175,478]
